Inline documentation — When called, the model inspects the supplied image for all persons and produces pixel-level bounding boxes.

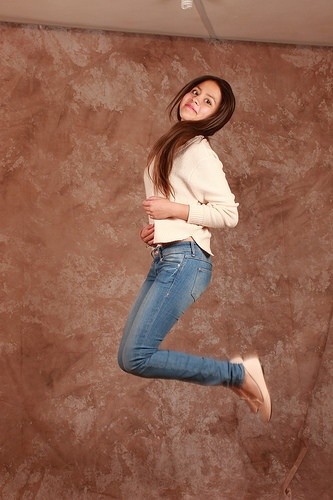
[115,75,275,423]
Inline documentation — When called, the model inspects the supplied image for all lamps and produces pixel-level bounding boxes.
[180,0,193,10]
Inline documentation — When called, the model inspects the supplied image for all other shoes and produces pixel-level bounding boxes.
[227,355,260,414]
[239,358,272,420]
